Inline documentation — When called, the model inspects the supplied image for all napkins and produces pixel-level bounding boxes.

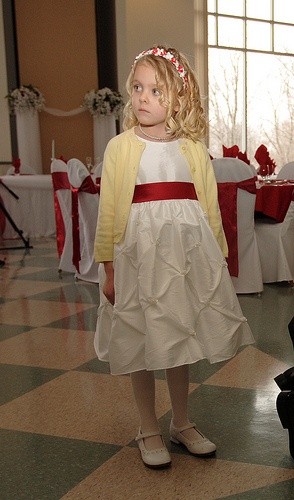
[12,159,22,174]
[254,144,277,176]
[238,152,250,164]
[222,146,239,158]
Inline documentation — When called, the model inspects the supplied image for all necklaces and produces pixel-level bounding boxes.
[138,123,174,140]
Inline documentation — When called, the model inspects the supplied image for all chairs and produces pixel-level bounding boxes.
[51,159,103,283]
[277,162,294,180]
[255,188,294,285]
[210,158,264,299]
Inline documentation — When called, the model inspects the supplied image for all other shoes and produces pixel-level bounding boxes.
[135,426,172,467]
[169,417,217,455]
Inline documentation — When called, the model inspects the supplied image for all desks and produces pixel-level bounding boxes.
[0,174,57,239]
[255,180,294,222]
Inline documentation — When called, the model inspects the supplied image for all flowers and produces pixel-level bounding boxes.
[5,84,45,117]
[81,86,126,119]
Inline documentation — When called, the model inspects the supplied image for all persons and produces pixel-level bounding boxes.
[95,46,257,470]
